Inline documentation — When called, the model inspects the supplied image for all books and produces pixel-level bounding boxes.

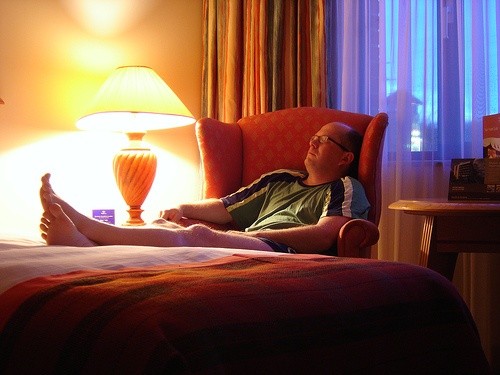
[482,114,500,159]
[448,158,500,203]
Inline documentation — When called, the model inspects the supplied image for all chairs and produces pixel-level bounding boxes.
[195,107,390,259]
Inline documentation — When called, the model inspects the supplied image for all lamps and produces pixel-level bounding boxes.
[78,66,196,225]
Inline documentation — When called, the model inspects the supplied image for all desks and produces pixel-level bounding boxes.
[388,197,499,375]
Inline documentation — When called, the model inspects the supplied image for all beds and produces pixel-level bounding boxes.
[0,241,492,375]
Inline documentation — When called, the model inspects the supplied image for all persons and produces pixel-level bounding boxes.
[39,121,371,254]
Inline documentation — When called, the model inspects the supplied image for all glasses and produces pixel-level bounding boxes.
[309,135,349,153]
